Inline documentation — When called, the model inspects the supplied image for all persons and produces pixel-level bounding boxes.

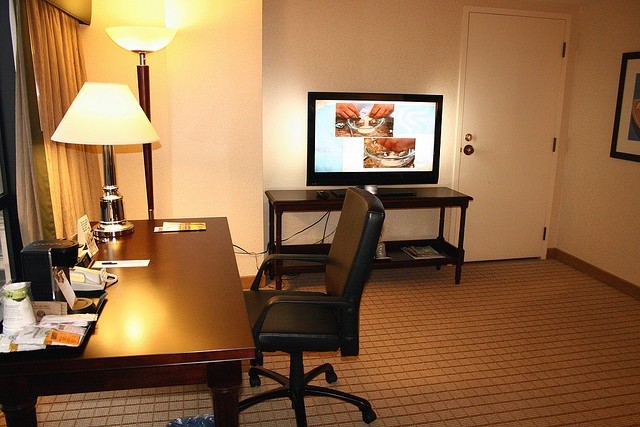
[379,138,415,152]
[336,103,394,119]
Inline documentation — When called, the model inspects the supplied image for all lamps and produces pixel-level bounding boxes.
[104,23,176,218]
[51,81,159,238]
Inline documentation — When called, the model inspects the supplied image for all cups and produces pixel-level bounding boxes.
[2,284,37,338]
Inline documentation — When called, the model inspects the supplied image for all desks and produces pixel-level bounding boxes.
[265,186,474,291]
[0,217,257,426]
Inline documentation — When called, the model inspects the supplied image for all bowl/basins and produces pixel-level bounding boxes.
[364,139,415,168]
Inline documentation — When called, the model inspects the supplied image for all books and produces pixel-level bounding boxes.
[400,244,446,259]
[161,221,207,232]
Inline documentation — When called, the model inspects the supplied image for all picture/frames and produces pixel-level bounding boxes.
[610,51,639,162]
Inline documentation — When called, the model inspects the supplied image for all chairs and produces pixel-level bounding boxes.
[237,187,385,426]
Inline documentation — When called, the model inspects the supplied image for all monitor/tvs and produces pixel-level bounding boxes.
[306,91,443,197]
